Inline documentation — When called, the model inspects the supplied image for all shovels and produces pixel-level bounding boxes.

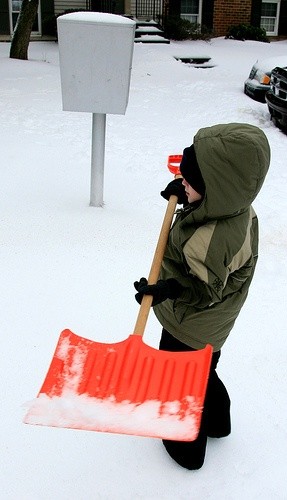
[24,154,213,441]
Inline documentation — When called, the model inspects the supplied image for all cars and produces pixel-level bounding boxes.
[244,57,287,136]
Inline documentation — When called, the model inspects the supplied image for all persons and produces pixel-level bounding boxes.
[131,123,270,472]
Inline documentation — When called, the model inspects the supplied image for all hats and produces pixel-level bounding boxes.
[179,144,206,197]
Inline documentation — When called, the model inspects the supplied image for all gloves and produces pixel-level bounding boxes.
[160,178,188,205]
[134,277,182,307]
